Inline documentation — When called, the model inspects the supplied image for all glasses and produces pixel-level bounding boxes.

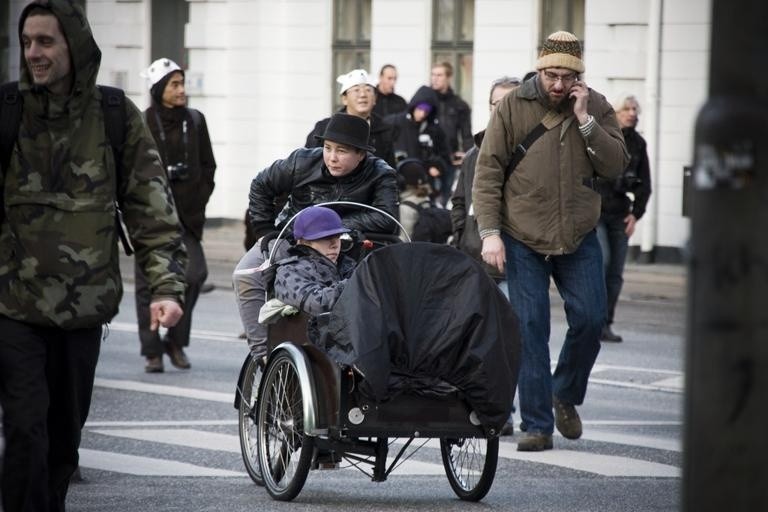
[492,78,520,85]
[542,68,577,80]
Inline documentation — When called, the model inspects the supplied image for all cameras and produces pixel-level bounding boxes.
[167,165,191,181]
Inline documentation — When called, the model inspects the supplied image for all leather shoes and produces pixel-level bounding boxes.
[145,356,162,372]
[162,335,192,368]
[601,324,623,342]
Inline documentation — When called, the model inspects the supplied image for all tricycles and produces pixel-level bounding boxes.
[235,198,520,501]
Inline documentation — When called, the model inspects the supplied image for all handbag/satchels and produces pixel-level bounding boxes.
[460,215,507,279]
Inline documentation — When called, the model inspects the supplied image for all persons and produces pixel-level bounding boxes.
[1,0,191,511]
[232,113,400,469]
[450,74,524,437]
[137,55,217,371]
[274,206,358,348]
[595,93,650,343]
[470,31,634,452]
[304,61,475,244]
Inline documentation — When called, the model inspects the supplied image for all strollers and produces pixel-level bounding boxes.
[388,156,457,250]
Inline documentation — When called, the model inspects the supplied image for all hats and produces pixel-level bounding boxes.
[536,31,586,73]
[314,114,376,154]
[295,207,351,241]
[336,70,380,96]
[139,58,182,91]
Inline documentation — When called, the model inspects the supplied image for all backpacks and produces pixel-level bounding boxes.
[400,198,452,244]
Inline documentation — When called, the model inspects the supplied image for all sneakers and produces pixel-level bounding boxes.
[552,393,582,439]
[517,432,552,451]
